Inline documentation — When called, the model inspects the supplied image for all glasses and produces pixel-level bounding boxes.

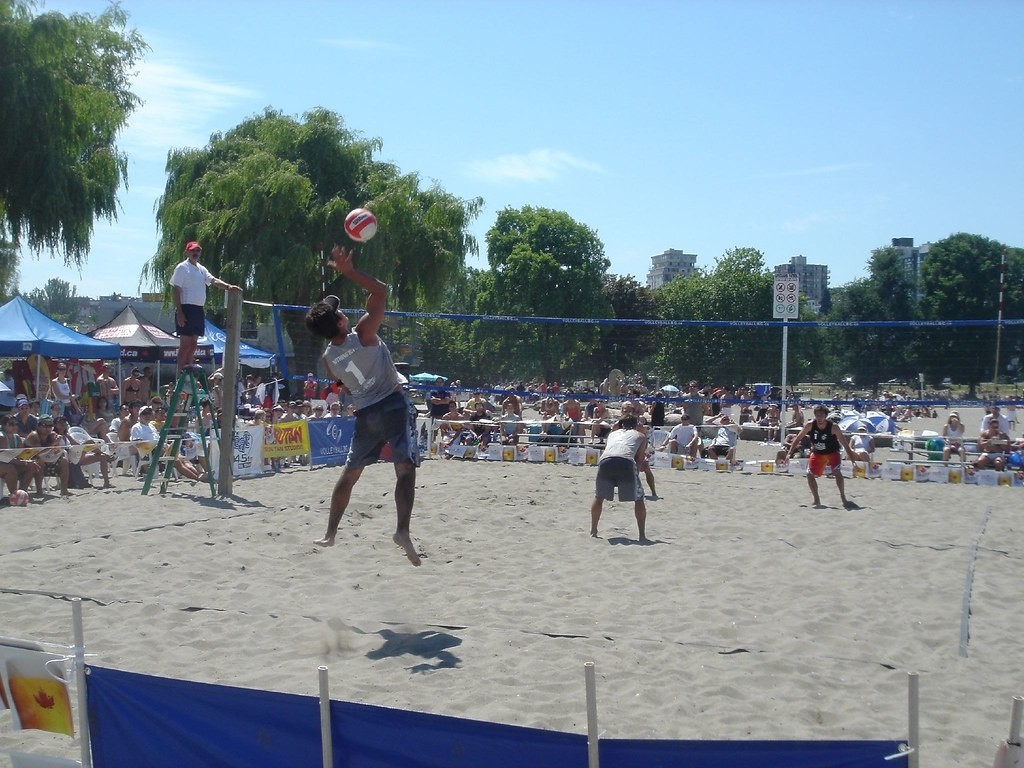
[4,422,19,427]
[722,418,729,422]
[990,426,999,429]
[318,409,324,412]
[154,401,162,405]
[40,422,54,428]
[142,410,151,415]
[132,372,140,377]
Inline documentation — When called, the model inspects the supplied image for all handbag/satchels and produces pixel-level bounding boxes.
[39,385,64,418]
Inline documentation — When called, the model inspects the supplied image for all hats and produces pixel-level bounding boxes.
[308,373,313,377]
[17,393,26,400]
[209,373,223,380]
[949,410,959,417]
[857,425,867,431]
[273,405,284,412]
[138,406,151,415]
[38,414,54,426]
[323,295,340,316]
[295,400,305,407]
[18,400,29,408]
[186,242,202,252]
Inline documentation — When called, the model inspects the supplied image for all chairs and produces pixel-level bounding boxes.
[0,427,179,499]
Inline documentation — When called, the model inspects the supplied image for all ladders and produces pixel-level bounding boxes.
[140,367,234,499]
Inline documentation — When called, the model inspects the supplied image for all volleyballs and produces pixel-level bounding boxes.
[343,207,377,242]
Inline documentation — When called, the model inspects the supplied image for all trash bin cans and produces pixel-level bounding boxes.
[925,439,945,461]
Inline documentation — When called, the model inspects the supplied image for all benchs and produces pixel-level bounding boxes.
[739,428,894,447]
[425,421,737,461]
[886,435,1024,471]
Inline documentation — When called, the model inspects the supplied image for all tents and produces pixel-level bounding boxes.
[0,296,278,411]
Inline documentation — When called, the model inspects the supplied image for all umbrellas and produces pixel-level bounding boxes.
[409,372,448,383]
[838,414,876,432]
[661,384,679,392]
[863,409,897,435]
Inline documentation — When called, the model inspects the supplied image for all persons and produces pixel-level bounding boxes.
[419,366,1024,497]
[170,242,241,369]
[306,246,420,569]
[0,363,357,499]
[781,403,860,509]
[592,416,651,541]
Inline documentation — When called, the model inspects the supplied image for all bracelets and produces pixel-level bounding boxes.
[226,284,230,289]
